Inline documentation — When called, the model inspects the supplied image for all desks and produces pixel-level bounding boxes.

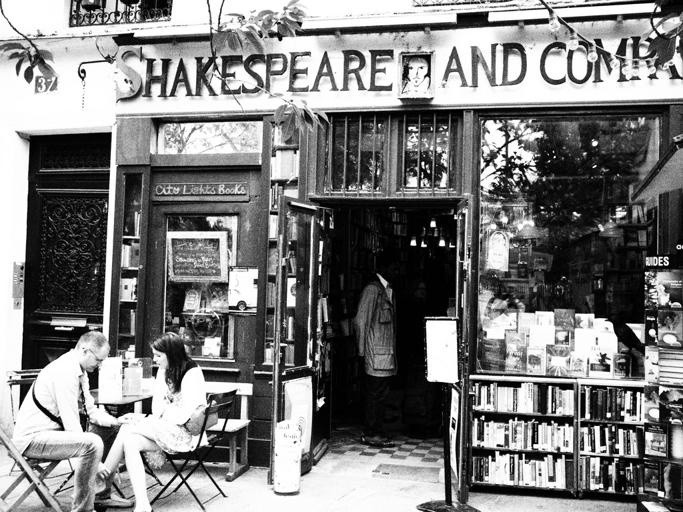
[86,389,164,501]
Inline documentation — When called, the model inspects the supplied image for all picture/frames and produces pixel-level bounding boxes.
[398,49,437,103]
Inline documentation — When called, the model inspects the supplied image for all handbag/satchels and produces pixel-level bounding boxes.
[186,399,218,435]
[58,411,86,433]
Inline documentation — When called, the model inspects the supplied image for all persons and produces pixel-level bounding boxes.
[354,259,400,443]
[95,332,208,512]
[8,328,137,512]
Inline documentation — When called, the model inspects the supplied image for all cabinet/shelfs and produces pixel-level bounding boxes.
[116,181,297,367]
[467,188,683,504]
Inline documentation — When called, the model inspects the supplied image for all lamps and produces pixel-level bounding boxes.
[539,0,683,78]
[409,217,456,251]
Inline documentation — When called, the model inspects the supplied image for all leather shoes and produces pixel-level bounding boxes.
[361,435,395,447]
[95,493,134,508]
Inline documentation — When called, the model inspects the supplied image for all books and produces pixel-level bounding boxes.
[470,306,682,502]
[120,211,140,358]
[50,316,87,327]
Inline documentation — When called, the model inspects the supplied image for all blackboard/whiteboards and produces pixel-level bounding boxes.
[171,238,221,276]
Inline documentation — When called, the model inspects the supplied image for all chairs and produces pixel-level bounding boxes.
[150,389,237,511]
[0,427,72,512]
[8,370,72,477]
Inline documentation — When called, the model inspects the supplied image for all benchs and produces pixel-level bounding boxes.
[207,381,253,481]
